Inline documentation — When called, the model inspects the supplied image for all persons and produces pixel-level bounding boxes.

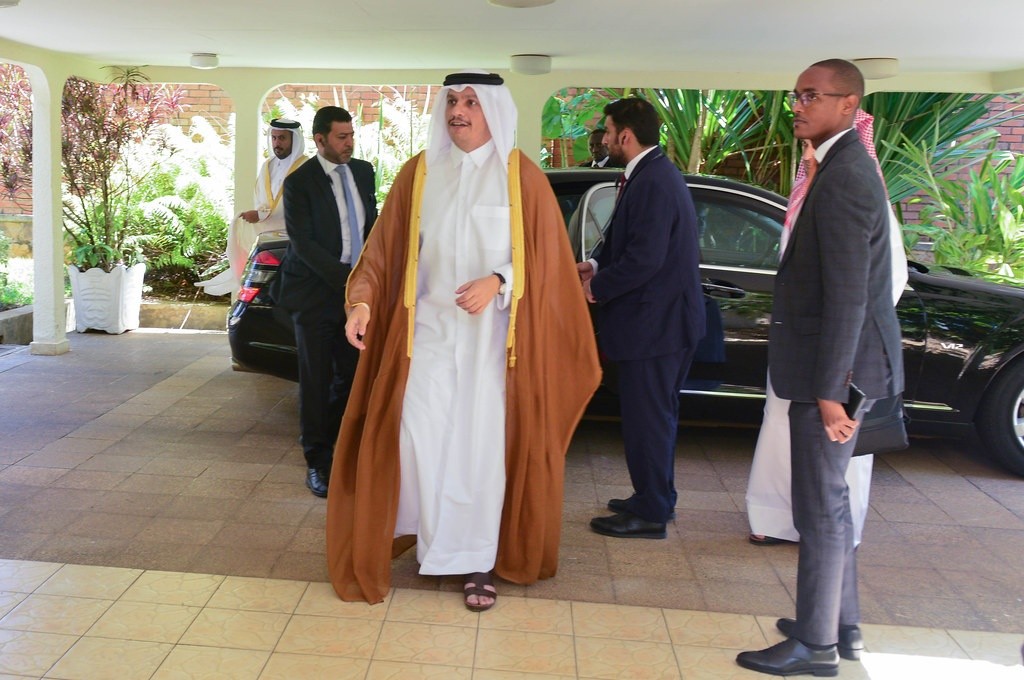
[579,128,626,168]
[283,106,378,498]
[737,59,911,676]
[238,118,309,236]
[325,69,603,613]
[575,97,708,540]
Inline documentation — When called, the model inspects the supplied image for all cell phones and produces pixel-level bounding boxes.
[841,382,866,421]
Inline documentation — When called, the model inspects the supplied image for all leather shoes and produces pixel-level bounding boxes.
[305,463,330,496]
[589,509,668,539]
[777,616,862,661]
[609,493,675,520]
[737,637,841,678]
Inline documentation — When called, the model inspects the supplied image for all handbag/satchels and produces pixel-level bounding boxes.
[852,394,909,457]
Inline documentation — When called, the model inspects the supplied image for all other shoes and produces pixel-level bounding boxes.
[749,532,788,543]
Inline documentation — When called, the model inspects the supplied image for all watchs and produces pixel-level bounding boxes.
[492,273,507,295]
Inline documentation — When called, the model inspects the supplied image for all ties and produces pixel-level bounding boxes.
[594,164,599,168]
[334,164,363,270]
[804,156,818,196]
[616,171,628,205]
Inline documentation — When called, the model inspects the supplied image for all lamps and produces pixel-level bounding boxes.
[847,58,898,80]
[510,55,551,75]
[190,53,219,69]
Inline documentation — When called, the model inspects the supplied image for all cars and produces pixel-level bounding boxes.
[227,169,1024,483]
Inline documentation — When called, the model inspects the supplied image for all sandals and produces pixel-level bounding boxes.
[463,571,497,611]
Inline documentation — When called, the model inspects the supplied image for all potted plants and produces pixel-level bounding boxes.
[0,61,191,334]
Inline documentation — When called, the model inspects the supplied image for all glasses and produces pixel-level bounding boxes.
[786,90,850,109]
